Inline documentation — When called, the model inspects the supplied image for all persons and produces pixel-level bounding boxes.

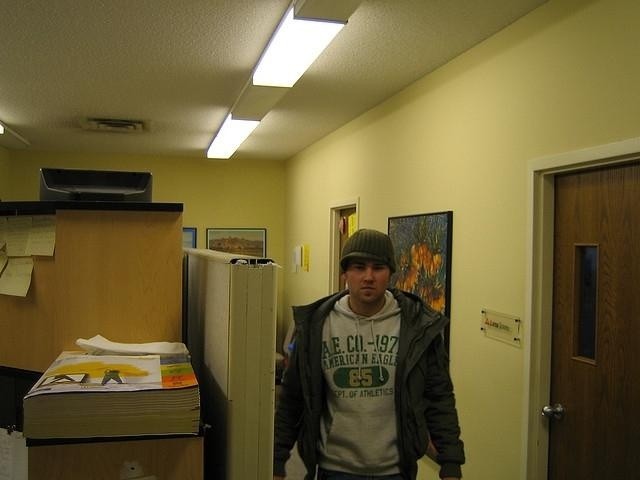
[272,228,465,480]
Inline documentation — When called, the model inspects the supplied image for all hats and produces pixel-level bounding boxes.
[339,228,396,276]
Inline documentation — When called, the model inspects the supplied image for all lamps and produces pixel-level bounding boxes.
[198,1,360,159]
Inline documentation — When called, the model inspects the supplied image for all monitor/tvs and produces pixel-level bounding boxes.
[39,167,153,203]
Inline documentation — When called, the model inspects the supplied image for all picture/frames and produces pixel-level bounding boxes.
[182,226,267,259]
[387,210,454,376]
